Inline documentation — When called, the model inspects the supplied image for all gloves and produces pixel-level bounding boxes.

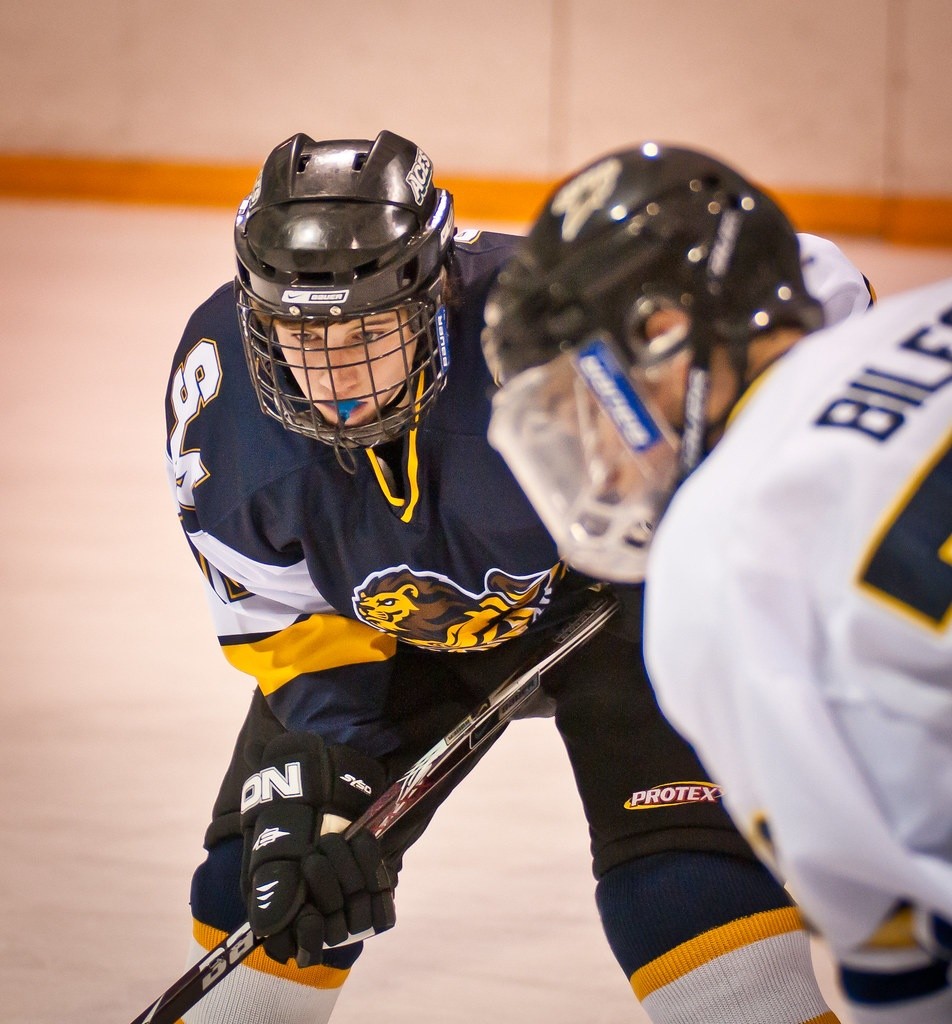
[238,734,397,962]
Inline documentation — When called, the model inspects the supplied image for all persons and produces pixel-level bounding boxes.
[160,124,852,1024]
[480,140,952,1024]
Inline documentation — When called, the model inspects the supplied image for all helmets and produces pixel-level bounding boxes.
[233,129,455,332]
[481,139,821,389]
